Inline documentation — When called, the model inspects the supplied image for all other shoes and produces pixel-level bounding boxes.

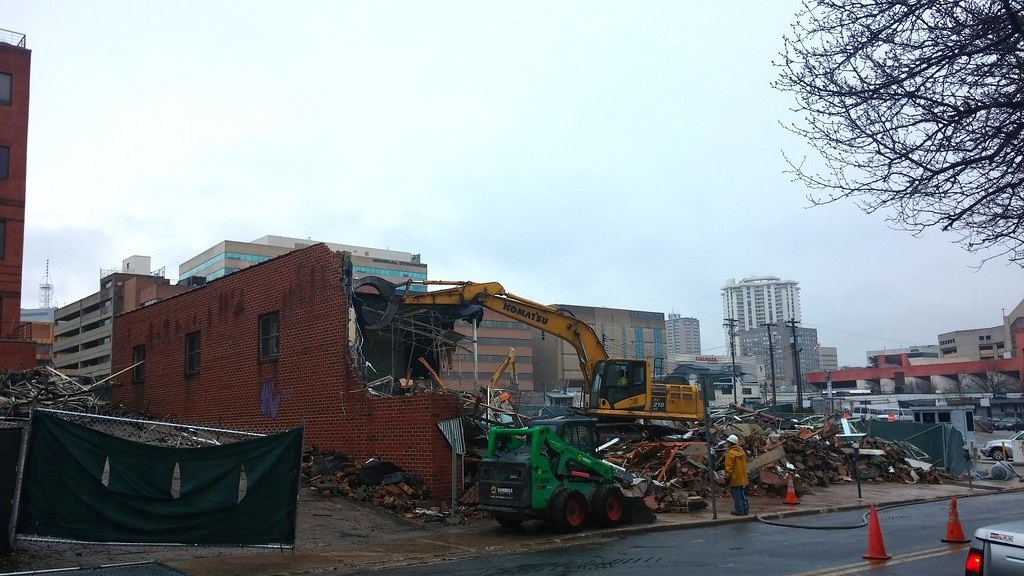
[731,511,744,516]
[744,512,749,515]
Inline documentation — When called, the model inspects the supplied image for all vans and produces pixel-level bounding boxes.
[877,407,914,423]
[852,404,881,422]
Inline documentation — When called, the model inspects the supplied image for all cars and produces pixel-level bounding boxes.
[973,414,1024,462]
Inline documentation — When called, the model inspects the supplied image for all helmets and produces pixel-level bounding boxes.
[726,434,738,445]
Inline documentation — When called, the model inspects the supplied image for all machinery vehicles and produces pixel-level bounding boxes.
[486,416,657,528]
[352,274,713,468]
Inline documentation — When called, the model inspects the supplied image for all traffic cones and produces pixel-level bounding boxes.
[785,473,803,504]
[863,502,891,561]
[940,496,971,543]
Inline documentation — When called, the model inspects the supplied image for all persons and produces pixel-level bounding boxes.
[722,434,750,517]
[617,368,629,387]
